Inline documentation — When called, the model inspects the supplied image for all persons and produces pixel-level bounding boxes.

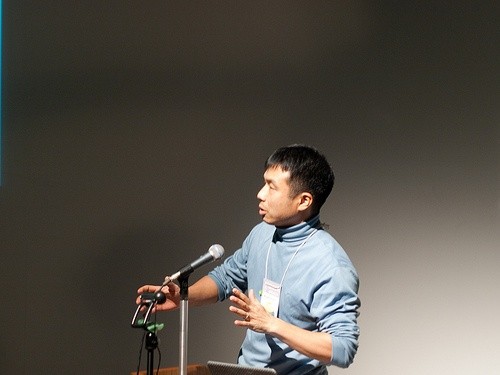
[136,143,360,372]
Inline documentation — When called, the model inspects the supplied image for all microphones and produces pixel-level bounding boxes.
[163,244,224,286]
